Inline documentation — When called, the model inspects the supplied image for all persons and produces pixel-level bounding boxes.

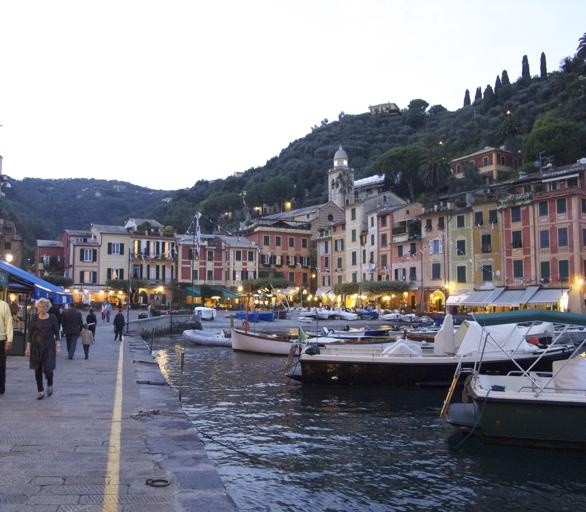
[78,323,93,360]
[85,309,97,339]
[0,297,13,394]
[22,297,60,400]
[60,302,83,359]
[112,310,124,341]
[56,306,63,329]
[105,301,112,323]
[101,301,106,320]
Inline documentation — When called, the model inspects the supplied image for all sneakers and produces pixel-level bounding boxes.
[46,387,53,397]
[36,392,45,400]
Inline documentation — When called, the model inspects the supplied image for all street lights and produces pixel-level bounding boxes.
[0,156,12,202]
